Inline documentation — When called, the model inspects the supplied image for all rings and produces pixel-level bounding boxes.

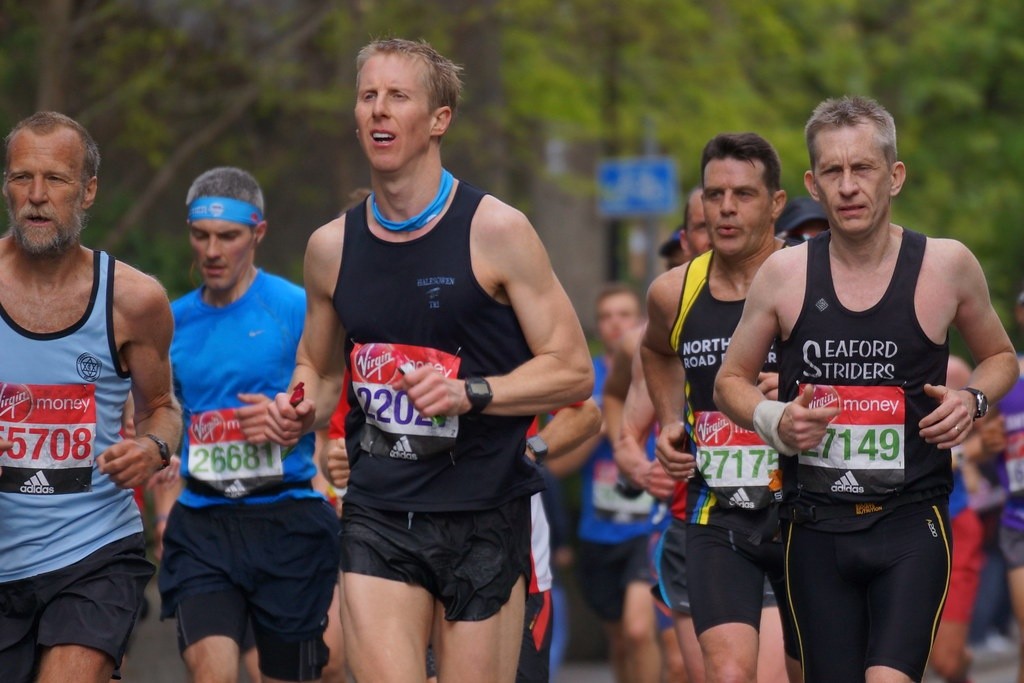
[954,425,963,433]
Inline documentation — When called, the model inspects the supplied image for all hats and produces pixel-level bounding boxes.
[777,198,828,237]
[660,227,685,256]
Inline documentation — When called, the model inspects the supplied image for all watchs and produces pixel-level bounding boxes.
[144,434,171,471]
[526,435,548,465]
[960,386,989,418]
[464,376,493,422]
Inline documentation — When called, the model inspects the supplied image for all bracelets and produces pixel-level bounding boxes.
[753,400,802,457]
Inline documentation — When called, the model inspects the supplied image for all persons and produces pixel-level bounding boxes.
[263,38,595,683]
[0,111,183,683]
[121,185,1024,683]
[712,95,1021,683]
[157,166,339,683]
[639,132,804,683]
